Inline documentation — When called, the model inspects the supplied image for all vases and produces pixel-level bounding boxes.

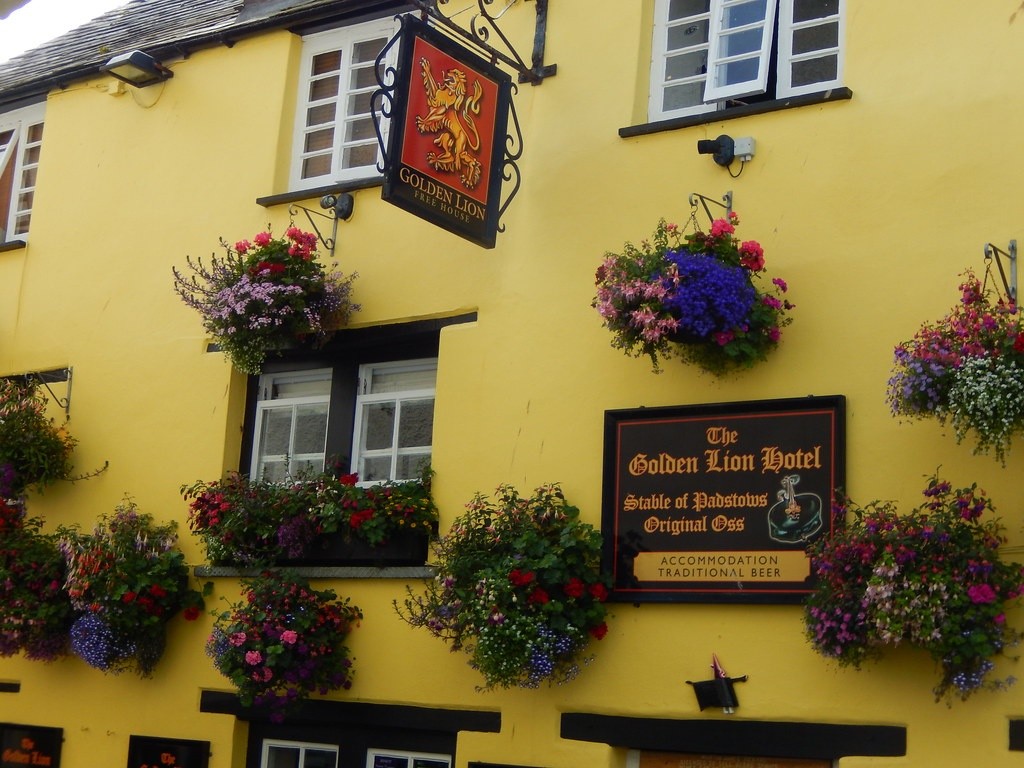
[318,537,429,564]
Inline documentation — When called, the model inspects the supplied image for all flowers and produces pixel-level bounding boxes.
[883,265,1023,469]
[0,462,67,665]
[0,378,108,499]
[181,452,438,567]
[51,491,214,681]
[205,571,363,722]
[173,223,362,376]
[800,465,1023,709]
[391,482,616,692]
[591,211,796,381]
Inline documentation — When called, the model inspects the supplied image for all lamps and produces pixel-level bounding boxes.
[98,51,174,90]
